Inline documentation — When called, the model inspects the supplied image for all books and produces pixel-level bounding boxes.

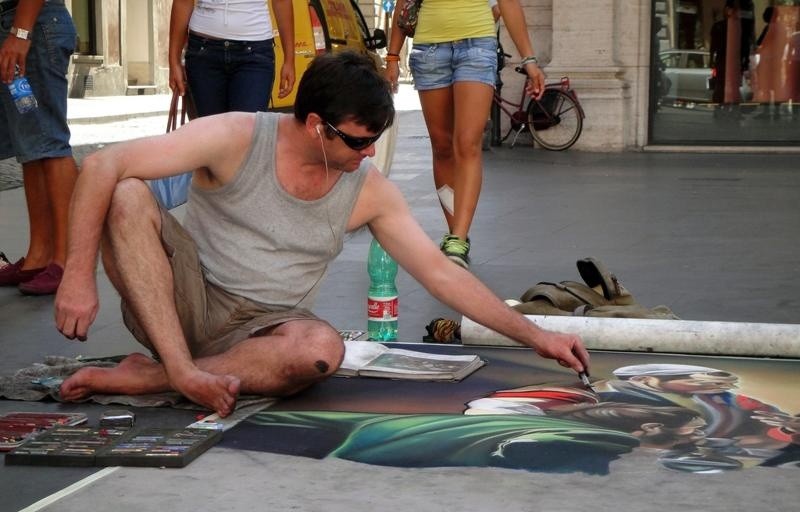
[332,335,487,385]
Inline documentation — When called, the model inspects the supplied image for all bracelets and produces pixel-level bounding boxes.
[522,55,538,66]
[385,52,401,62]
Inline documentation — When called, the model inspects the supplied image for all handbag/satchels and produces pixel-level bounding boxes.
[146,87,194,209]
[397,0,420,36]
[509,257,678,320]
[707,77,715,90]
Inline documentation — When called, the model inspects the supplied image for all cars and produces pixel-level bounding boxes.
[658,49,751,104]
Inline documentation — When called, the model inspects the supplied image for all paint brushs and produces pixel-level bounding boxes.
[12,427,126,454]
[0,413,81,442]
[112,434,200,457]
[580,371,595,393]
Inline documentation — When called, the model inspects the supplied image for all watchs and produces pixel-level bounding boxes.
[4,24,32,42]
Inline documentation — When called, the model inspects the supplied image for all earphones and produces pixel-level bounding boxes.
[315,123,321,136]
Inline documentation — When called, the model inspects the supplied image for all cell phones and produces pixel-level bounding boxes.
[100,409,136,428]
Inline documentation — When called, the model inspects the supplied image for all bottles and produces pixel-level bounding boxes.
[367,235,402,341]
[5,64,40,115]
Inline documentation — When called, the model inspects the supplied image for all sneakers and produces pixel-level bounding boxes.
[20,264,63,295]
[1,256,41,286]
[444,236,470,269]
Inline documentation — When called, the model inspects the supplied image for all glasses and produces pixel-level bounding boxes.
[327,121,386,151]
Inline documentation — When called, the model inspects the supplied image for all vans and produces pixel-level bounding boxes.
[260,0,394,125]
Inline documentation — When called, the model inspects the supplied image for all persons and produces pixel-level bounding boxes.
[656,10,671,110]
[785,21,800,116]
[754,4,782,109]
[49,43,594,421]
[705,2,750,104]
[161,0,298,118]
[378,1,550,269]
[0,1,82,298]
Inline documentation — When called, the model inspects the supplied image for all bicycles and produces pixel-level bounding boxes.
[484,51,584,149]
